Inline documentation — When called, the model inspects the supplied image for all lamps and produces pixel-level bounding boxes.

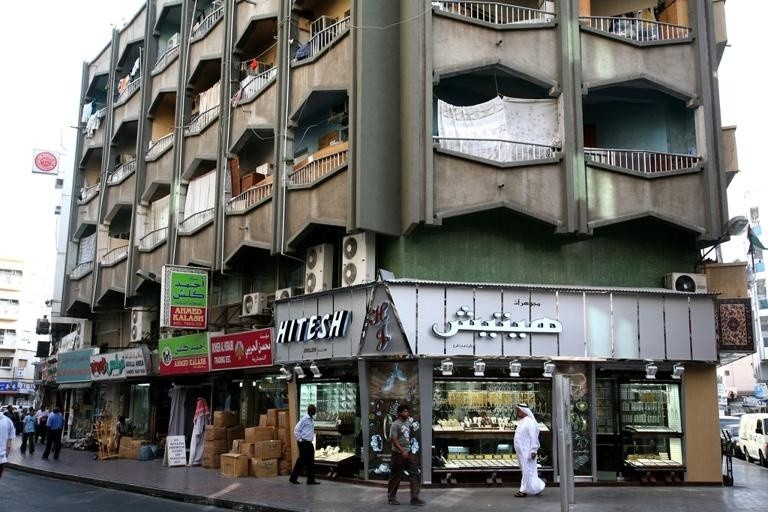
[436,356,556,378]
[644,362,685,379]
[280,360,322,382]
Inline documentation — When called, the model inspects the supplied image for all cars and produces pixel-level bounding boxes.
[718,384,768,468]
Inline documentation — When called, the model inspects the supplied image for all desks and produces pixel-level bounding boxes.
[313,450,358,477]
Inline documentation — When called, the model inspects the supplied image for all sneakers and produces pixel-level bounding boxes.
[410,498,425,506]
[288,478,300,485]
[307,481,321,485]
[21,450,60,460]
[514,492,527,498]
[388,499,400,505]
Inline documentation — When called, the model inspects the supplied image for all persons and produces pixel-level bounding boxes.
[513,404,546,497]
[288,404,321,485]
[0,411,16,480]
[387,404,426,505]
[3,402,65,459]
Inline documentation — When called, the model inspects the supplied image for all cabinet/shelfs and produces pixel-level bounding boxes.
[432,420,553,485]
[620,424,687,485]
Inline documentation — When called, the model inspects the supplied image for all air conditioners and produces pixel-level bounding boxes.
[242,292,267,316]
[342,230,376,287]
[274,286,295,301]
[665,272,709,293]
[130,309,152,342]
[303,242,333,295]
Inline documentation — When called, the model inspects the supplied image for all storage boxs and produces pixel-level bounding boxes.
[203,408,292,478]
[117,437,149,459]
